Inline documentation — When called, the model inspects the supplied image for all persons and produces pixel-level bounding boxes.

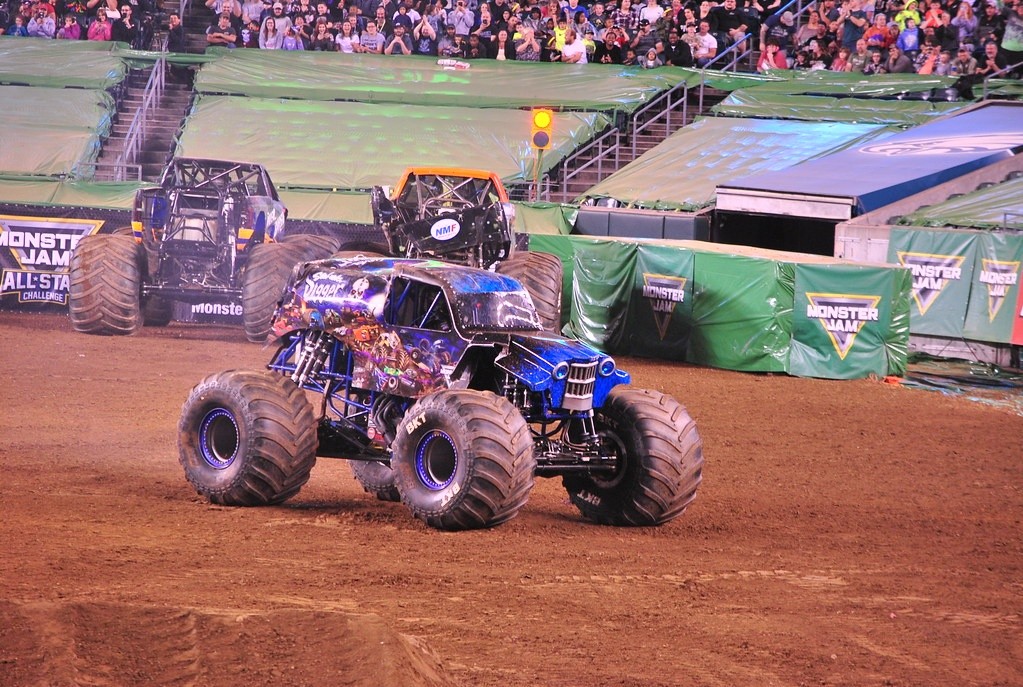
[310,21,334,51]
[0,0,149,44]
[462,34,486,58]
[168,13,185,53]
[486,28,516,61]
[863,50,886,75]
[384,22,414,55]
[753,39,788,75]
[759,10,798,51]
[561,28,587,64]
[413,14,438,55]
[515,27,540,61]
[259,17,283,49]
[207,11,237,48]
[334,19,360,53]
[205,0,783,68]
[791,0,1023,79]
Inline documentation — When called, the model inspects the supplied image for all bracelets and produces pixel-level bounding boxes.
[849,15,851,18]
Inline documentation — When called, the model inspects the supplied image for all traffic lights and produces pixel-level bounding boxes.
[530,107,553,148]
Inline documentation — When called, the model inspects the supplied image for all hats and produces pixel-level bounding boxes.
[220,11,231,19]
[783,11,794,26]
[958,46,967,52]
[263,0,272,4]
[687,22,696,28]
[985,1,996,7]
[38,4,47,10]
[584,29,594,35]
[393,22,406,31]
[273,2,284,9]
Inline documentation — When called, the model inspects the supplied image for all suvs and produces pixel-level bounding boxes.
[176,252,706,528]
[294,166,563,339]
[68,156,342,343]
